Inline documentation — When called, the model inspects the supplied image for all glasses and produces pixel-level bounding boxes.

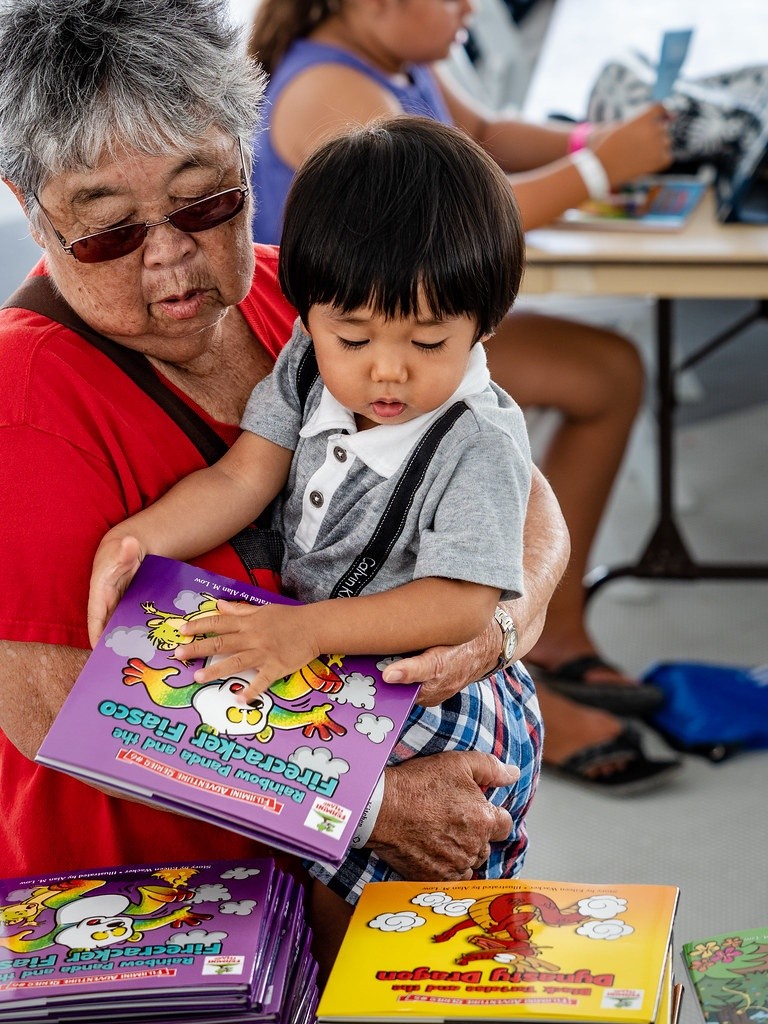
[32,135,251,264]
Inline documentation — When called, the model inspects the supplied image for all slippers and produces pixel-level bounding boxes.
[542,719,683,795]
[521,652,673,716]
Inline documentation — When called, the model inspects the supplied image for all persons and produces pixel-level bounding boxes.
[244,0,683,793]
[0,1,570,982]
[88,115,543,982]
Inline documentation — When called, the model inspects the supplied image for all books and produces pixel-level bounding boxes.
[0,554,768,1024]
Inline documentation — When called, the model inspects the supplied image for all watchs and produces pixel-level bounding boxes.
[477,606,518,682]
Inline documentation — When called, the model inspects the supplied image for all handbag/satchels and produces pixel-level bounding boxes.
[638,659,768,761]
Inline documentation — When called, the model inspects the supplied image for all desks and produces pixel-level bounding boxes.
[513,186,768,616]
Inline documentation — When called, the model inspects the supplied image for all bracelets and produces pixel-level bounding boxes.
[572,147,612,200]
[571,121,590,155]
